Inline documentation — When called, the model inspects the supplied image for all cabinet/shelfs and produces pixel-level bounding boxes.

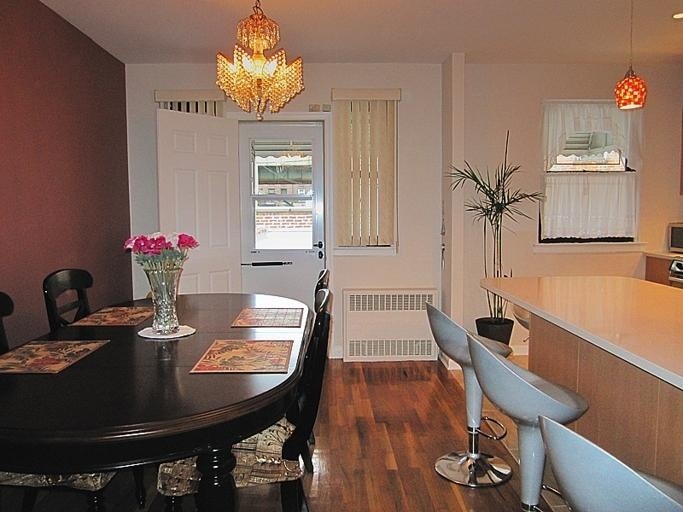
[644,253,683,289]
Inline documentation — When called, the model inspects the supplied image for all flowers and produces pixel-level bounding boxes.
[123,232,200,325]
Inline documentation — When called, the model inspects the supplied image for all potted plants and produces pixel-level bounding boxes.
[443,129,543,345]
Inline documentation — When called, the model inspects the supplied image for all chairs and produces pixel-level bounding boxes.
[156,285,334,512]
[421,301,518,487]
[43,268,94,335]
[231,268,329,463]
[532,414,683,511]
[460,328,588,512]
[0,286,146,512]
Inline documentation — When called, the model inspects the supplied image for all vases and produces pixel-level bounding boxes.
[134,264,186,338]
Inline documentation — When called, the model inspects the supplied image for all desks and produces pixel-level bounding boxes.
[0,293,331,508]
[477,275,683,510]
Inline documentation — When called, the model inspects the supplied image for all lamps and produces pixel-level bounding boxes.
[215,2,305,121]
[613,2,647,112]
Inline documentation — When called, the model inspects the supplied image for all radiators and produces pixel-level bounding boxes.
[341,288,439,362]
